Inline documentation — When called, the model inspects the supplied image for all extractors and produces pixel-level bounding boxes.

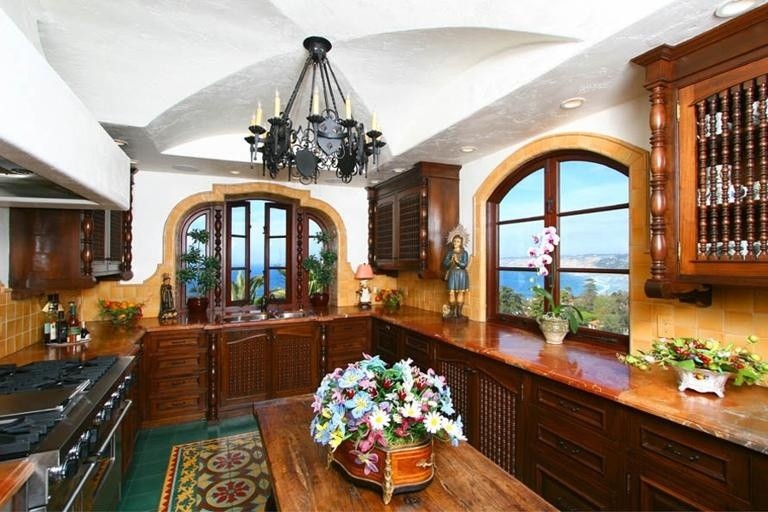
[0,10,133,214]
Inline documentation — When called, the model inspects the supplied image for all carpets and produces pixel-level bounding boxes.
[154,428,276,512]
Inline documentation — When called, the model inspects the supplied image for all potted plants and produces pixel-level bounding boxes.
[178,228,218,310]
[301,231,338,306]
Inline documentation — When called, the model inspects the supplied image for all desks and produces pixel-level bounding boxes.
[249,385,566,512]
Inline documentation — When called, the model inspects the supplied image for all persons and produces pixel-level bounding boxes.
[444,224,470,318]
[160,272,176,313]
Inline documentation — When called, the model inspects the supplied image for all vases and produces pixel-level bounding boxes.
[325,425,442,504]
[670,360,732,397]
[536,309,569,343]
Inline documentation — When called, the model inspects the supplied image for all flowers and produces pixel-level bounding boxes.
[526,226,584,332]
[616,334,768,388]
[310,350,470,471]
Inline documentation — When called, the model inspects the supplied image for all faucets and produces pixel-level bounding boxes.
[260,296,268,312]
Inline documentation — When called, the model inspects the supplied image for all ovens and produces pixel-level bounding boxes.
[48,398,134,510]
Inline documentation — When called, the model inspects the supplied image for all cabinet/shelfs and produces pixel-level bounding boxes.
[632,3,767,309]
[8,164,136,294]
[437,342,525,481]
[524,372,623,511]
[121,341,143,480]
[143,328,206,422]
[365,162,462,279]
[214,325,324,413]
[325,316,371,372]
[373,319,436,375]
[622,400,768,511]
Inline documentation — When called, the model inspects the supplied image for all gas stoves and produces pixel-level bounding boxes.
[1,357,140,512]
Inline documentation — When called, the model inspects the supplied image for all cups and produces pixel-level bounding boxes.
[698,97,767,257]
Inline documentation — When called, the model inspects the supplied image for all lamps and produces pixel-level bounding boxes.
[243,36,387,187]
[354,263,374,303]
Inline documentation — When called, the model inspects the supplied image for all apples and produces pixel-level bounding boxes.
[104,301,138,309]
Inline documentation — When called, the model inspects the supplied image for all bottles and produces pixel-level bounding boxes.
[44,345,88,361]
[42,293,90,341]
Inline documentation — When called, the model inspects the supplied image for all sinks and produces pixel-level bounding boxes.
[269,310,319,321]
[218,312,276,323]
